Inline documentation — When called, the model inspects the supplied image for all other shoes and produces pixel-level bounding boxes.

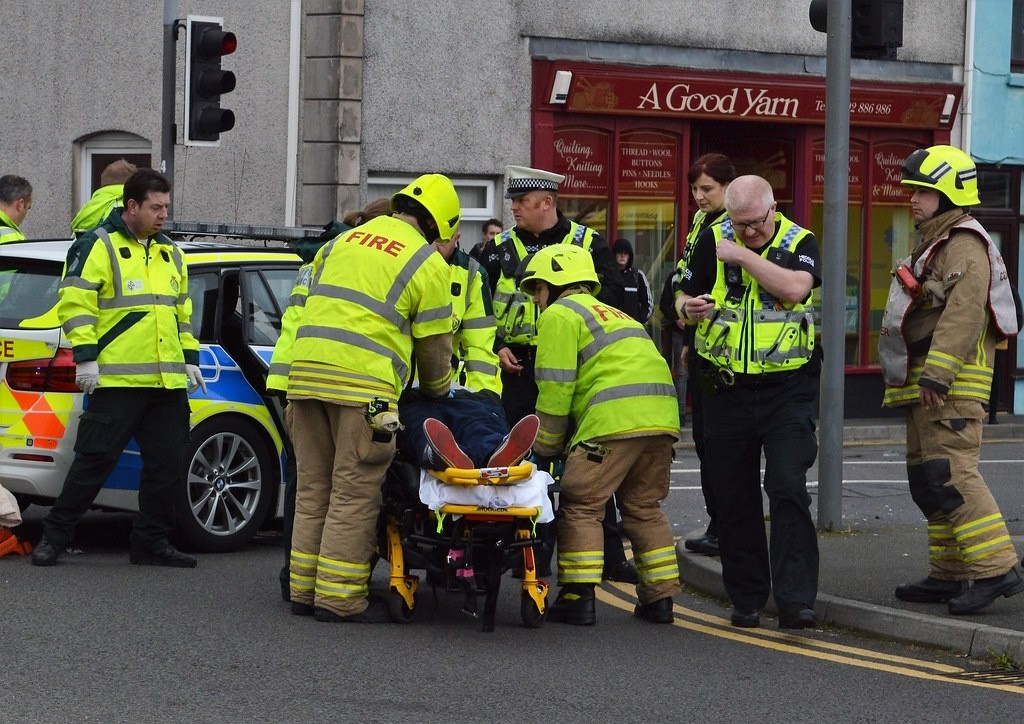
[602,561,638,584]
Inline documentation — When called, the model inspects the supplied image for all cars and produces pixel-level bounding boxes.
[0,220,327,552]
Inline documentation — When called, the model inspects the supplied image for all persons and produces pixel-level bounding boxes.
[32,167,207,567]
[0,175,32,304]
[676,175,822,630]
[266,165,681,625]
[878,145,1024,615]
[71,159,138,238]
[660,154,737,555]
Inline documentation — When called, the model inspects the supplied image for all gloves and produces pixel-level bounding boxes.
[75,361,100,394]
[185,364,207,395]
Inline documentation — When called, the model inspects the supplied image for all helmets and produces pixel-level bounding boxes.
[900,145,981,206]
[520,244,601,297]
[389,174,462,242]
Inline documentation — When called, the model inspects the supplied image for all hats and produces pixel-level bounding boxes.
[505,165,566,198]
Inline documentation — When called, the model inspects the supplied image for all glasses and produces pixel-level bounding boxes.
[729,206,770,231]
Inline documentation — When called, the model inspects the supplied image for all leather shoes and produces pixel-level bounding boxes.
[731,608,759,628]
[896,575,968,603]
[948,563,1024,615]
[778,603,817,629]
[686,534,720,556]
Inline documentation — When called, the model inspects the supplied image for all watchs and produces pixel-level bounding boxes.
[680,303,689,320]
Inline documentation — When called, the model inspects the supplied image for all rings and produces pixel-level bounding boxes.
[697,312,700,318]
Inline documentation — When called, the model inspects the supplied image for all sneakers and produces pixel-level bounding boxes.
[487,414,540,468]
[291,591,392,623]
[633,596,674,622]
[31,539,61,566]
[549,585,596,625]
[130,546,197,569]
[423,418,474,470]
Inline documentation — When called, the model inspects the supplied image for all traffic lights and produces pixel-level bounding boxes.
[809,0,828,35]
[185,14,238,148]
